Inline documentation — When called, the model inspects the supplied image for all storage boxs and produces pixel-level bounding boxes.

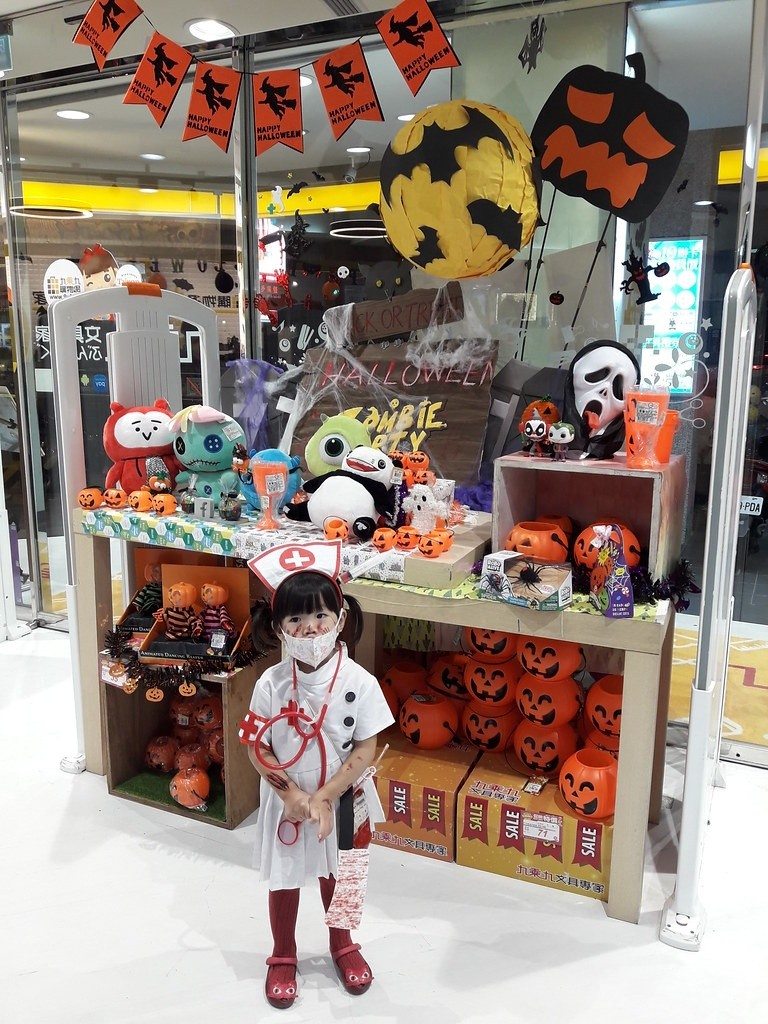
[492,449,685,582]
[100,546,282,831]
[368,724,613,902]
[479,550,572,610]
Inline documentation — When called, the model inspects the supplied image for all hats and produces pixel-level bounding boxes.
[247,539,343,612]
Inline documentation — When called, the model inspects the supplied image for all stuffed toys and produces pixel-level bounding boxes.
[103,400,395,537]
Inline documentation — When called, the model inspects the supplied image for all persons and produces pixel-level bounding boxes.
[240,570,395,1008]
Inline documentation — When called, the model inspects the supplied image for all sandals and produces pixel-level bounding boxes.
[332,943,372,996]
[265,954,297,1009]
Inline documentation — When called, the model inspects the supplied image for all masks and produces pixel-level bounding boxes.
[278,608,343,670]
